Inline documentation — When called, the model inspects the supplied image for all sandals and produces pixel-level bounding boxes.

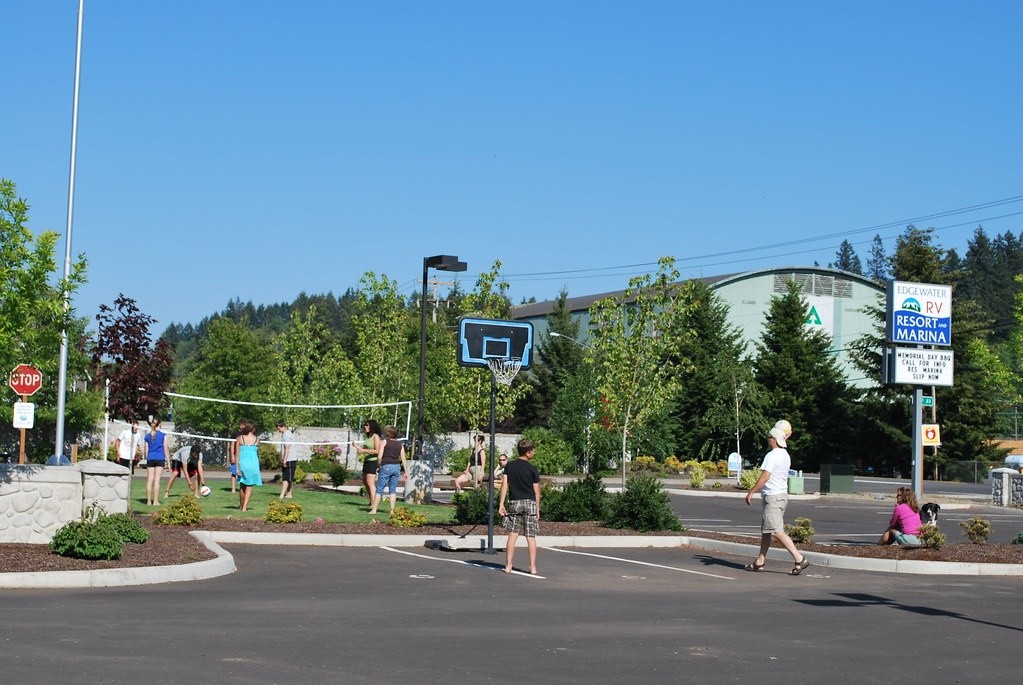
[789,558,809,575]
[745,559,765,572]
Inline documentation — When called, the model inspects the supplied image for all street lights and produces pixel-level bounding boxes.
[417,255,468,459]
[550,332,593,475]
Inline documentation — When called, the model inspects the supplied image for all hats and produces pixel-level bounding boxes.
[769,428,787,447]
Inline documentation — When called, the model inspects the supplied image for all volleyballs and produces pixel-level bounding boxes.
[200,485,211,496]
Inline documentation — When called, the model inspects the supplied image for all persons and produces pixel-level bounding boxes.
[878,487,925,545]
[494,454,508,479]
[117,421,142,474]
[230,419,247,493]
[235,420,263,511]
[352,419,382,507]
[368,425,409,514]
[144,419,170,505]
[499,439,542,574]
[164,445,206,499]
[455,435,486,491]
[745,428,810,576]
[275,420,297,499]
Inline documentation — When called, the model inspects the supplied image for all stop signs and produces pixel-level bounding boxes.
[9,364,43,395]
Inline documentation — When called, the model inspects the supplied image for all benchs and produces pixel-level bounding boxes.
[436,472,556,492]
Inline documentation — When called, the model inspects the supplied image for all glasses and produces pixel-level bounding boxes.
[896,494,902,498]
[768,436,773,439]
[499,458,506,461]
[365,424,370,426]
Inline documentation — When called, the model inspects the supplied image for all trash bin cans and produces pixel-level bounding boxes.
[820,464,856,493]
[788,477,805,494]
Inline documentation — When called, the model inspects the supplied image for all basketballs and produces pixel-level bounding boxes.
[775,419,792,436]
